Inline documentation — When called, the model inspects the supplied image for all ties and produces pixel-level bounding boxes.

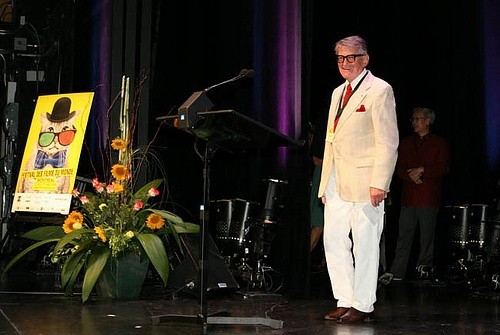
[334,84,352,133]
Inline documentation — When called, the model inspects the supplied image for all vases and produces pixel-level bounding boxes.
[98,247,150,298]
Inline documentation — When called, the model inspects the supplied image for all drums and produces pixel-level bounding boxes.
[445,205,472,251]
[469,204,496,250]
[254,177,290,225]
[208,198,260,253]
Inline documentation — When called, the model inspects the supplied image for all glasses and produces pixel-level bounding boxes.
[33,125,76,147]
[335,54,364,63]
[410,117,426,122]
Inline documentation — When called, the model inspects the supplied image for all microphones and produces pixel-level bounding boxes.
[204,68,256,93]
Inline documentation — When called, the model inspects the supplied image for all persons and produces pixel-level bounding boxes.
[317,35,400,324]
[310,133,326,271]
[383,108,450,287]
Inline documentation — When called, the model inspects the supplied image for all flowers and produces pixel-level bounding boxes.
[2,135,202,303]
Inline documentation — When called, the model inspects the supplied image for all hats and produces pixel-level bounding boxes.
[45,97,76,122]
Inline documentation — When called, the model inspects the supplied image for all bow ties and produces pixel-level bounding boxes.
[35,150,67,169]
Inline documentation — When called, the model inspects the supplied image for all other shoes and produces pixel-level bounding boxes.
[322,307,375,323]
[392,276,403,280]
